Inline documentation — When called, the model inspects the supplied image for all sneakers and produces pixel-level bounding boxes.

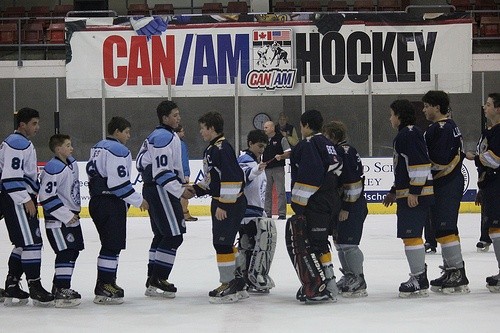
[93,277,124,304]
[336,268,368,298]
[4,274,30,307]
[486,267,500,292]
[296,281,337,303]
[0,288,6,302]
[144,277,177,298]
[234,270,271,296]
[398,263,429,299]
[429,261,471,296]
[424,242,436,255]
[208,277,250,304]
[324,276,338,298]
[477,240,491,253]
[27,276,56,308]
[51,285,81,308]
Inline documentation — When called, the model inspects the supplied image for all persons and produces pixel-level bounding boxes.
[261,121,291,220]
[413,90,470,295]
[186,111,249,304]
[236,128,278,294]
[275,112,299,149]
[466,93,500,293]
[284,108,344,304]
[176,127,198,221]
[383,99,435,299]
[0,107,56,309]
[322,121,369,297]
[37,134,85,308]
[86,117,149,305]
[137,100,195,298]
[475,120,493,253]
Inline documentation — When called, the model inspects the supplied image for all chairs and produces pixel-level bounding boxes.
[152,4,174,15]
[0,5,73,44]
[201,3,224,13]
[274,0,500,46]
[127,4,149,15]
[227,2,247,13]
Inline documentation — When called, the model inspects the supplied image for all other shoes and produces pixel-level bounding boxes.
[184,210,198,221]
[278,216,286,219]
[268,215,272,218]
